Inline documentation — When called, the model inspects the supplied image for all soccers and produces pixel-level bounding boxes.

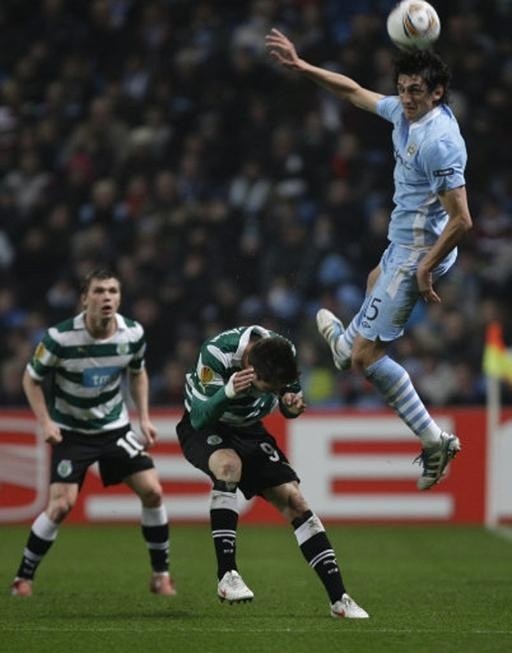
[387,0,441,55]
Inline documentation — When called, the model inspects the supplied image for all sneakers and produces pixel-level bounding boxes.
[151,571,174,595]
[216,571,254,605]
[315,308,353,370]
[12,575,31,595]
[416,431,461,491]
[329,595,368,619]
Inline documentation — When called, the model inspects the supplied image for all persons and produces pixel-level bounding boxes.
[176,323,372,618]
[10,267,176,597]
[263,25,476,492]
[0,0,512,408]
